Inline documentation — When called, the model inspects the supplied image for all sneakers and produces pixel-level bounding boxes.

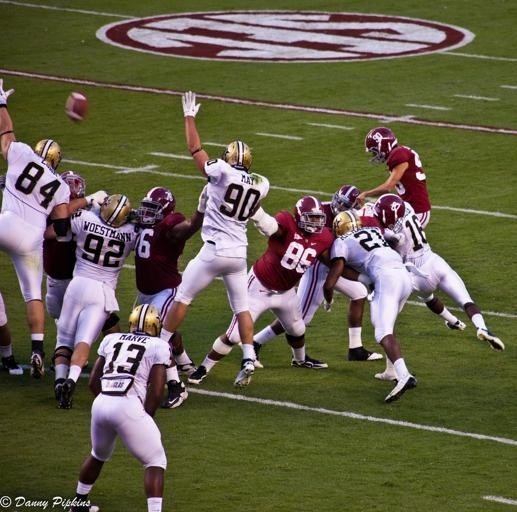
[30,350,47,377]
[373,357,417,402]
[71,500,102,511]
[177,359,211,385]
[290,351,330,371]
[238,338,264,368]
[444,316,468,332]
[232,359,255,388]
[348,344,385,363]
[54,376,78,410]
[1,350,23,373]
[165,377,189,409]
[475,326,505,351]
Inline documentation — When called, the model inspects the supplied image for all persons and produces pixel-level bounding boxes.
[68,304,172,511]
[0,77,509,410]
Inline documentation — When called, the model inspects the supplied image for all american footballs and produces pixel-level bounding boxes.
[64,91,88,123]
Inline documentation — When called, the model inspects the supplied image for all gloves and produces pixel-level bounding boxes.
[82,190,109,208]
[197,181,211,215]
[181,90,203,119]
[323,295,338,314]
[0,77,16,109]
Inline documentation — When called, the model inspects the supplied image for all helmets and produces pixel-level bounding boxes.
[128,303,166,337]
[99,193,134,229]
[294,194,327,233]
[55,170,86,204]
[222,140,254,172]
[331,209,362,236]
[372,192,406,227]
[330,184,361,213]
[364,125,398,162]
[34,139,63,171]
[140,185,175,227]
